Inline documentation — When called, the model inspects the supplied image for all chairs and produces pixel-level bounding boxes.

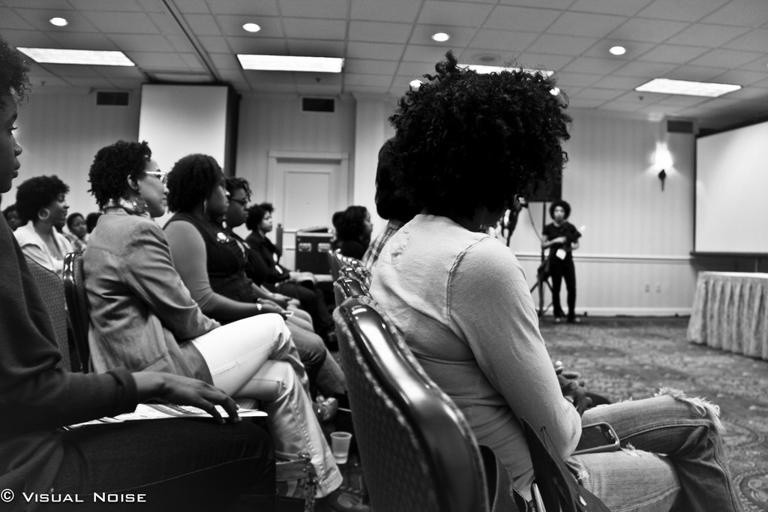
[24,251,625,512]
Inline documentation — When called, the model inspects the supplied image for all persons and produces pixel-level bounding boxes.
[1,36,741,511]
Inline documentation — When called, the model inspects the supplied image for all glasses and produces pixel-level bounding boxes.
[227,197,249,207]
[141,169,168,184]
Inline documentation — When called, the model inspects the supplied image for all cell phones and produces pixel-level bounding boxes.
[571,422,620,455]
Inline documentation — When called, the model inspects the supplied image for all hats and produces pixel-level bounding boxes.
[550,201,571,220]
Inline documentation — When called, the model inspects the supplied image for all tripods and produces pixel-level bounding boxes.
[530,202,567,318]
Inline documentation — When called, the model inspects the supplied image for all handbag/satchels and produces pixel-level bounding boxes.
[536,258,551,281]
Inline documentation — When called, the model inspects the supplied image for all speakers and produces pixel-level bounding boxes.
[525,171,561,201]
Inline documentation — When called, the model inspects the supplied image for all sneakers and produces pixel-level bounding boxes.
[554,315,580,324]
[320,397,340,422]
[312,488,369,511]
